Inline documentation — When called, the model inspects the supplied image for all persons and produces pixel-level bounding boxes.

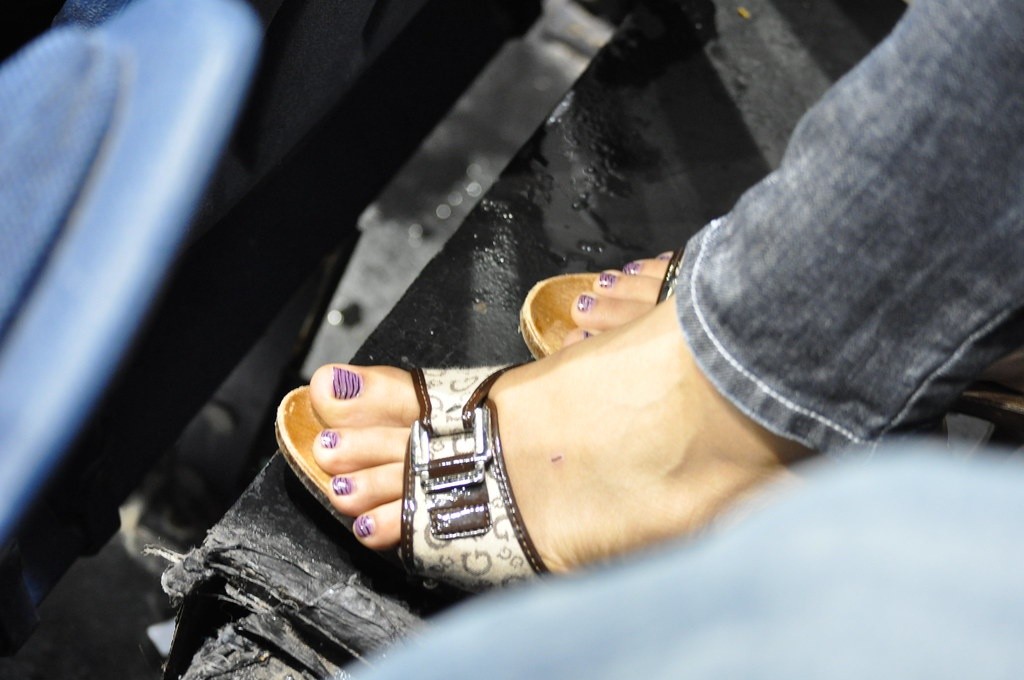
[276,0,1024,596]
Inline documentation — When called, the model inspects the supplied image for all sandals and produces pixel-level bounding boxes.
[274,358,554,595]
[519,242,1021,451]
